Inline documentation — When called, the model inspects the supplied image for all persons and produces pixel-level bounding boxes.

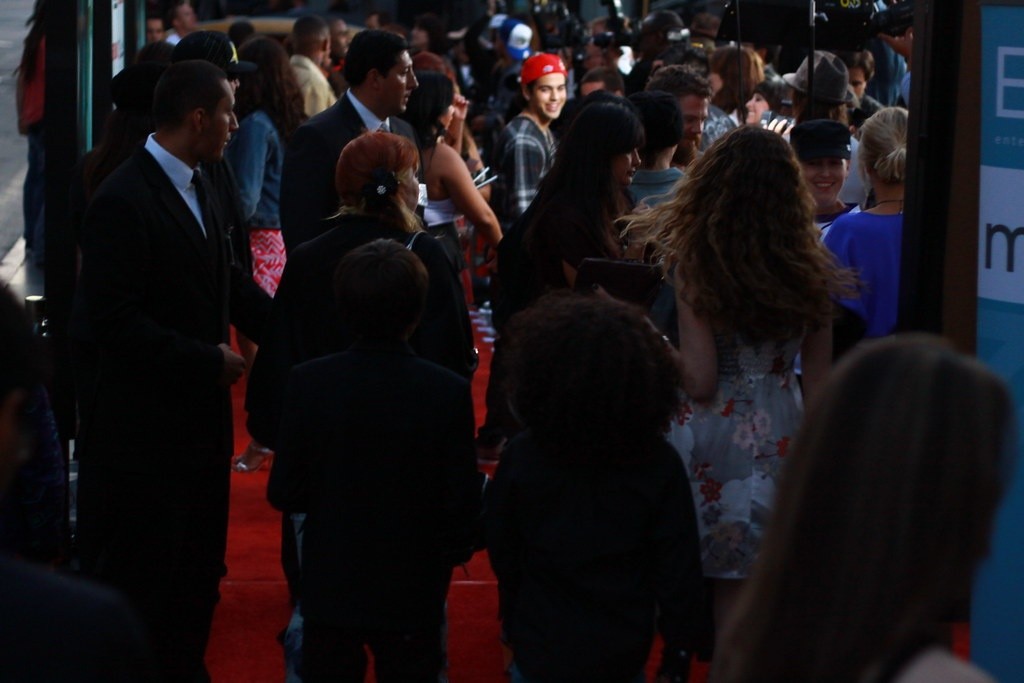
[716,331,1010,683]
[0,0,912,683]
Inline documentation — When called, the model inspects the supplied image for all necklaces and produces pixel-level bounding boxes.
[872,199,904,207]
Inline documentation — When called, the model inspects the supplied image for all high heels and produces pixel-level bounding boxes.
[233,437,272,473]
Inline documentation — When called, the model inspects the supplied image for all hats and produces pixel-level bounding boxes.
[521,52,568,84]
[789,117,851,161]
[488,14,508,28]
[170,30,258,77]
[501,17,534,58]
[782,49,855,104]
[754,73,789,116]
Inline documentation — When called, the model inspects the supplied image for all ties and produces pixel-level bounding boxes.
[192,170,215,246]
[379,123,388,133]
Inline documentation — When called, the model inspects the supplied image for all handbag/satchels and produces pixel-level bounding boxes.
[575,256,669,309]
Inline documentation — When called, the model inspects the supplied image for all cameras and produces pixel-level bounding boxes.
[593,0,644,55]
